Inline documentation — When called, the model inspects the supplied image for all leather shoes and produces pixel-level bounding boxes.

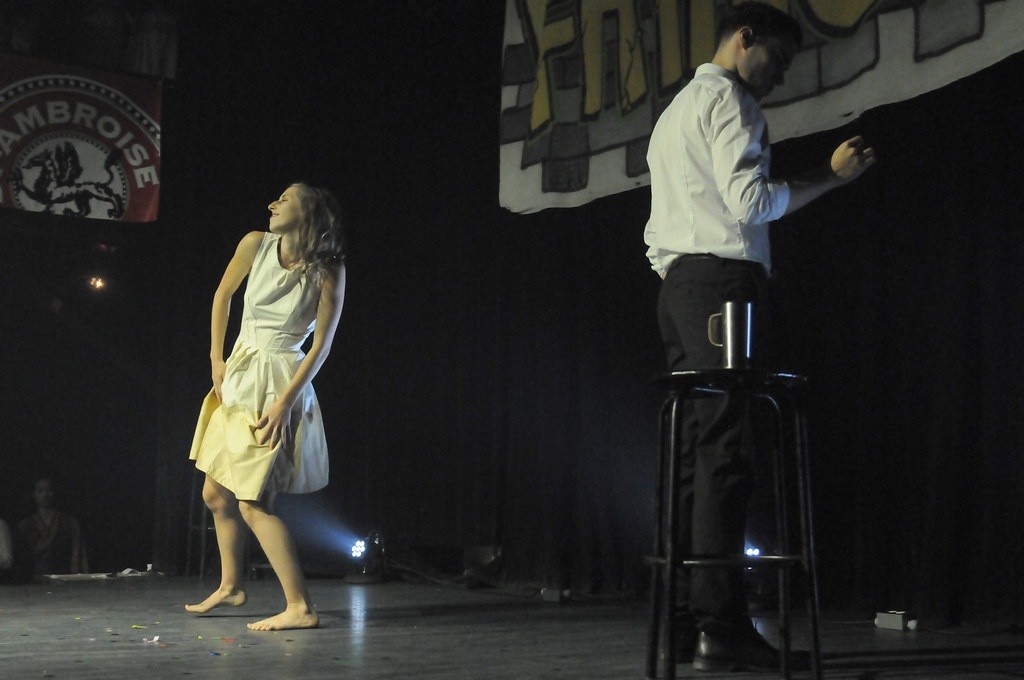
[693,627,814,673]
[658,625,700,662]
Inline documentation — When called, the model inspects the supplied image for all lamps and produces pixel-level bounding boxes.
[344,529,390,583]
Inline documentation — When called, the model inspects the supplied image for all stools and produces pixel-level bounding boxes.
[644,369,821,680]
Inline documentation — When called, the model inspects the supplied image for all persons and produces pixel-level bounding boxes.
[639,2,874,672]
[12,470,89,583]
[181,177,351,631]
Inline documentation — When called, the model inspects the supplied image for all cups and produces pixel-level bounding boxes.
[708,300,753,370]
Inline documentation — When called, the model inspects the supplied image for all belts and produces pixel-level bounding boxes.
[667,254,760,266]
